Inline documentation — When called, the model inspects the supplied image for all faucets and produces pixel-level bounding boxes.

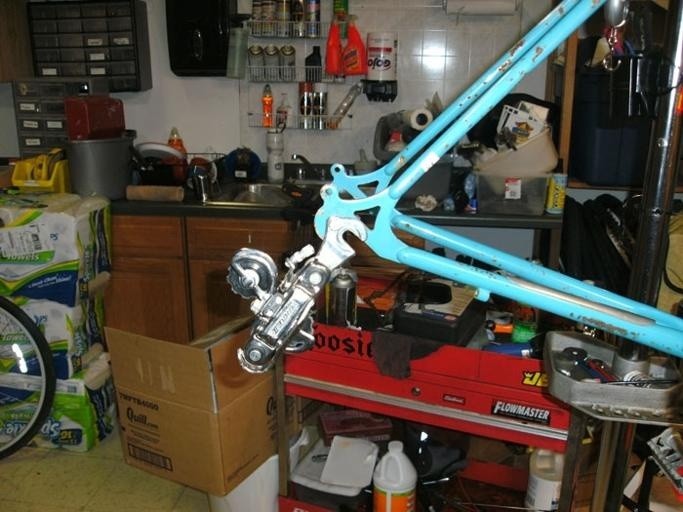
[292,152,319,179]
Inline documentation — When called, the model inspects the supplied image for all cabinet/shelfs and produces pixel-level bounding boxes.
[11,2,154,163]
[278,326,569,511]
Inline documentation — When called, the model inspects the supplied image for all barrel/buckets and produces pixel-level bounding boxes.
[64,128,138,200]
[523,447,567,512]
[371,441,418,512]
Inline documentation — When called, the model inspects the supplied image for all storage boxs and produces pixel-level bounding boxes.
[103,320,277,495]
[102,217,191,343]
[182,216,297,346]
[299,221,417,273]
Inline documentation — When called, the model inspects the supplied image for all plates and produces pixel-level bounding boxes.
[135,142,185,163]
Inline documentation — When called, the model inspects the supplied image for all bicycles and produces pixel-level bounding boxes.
[0,295,56,461]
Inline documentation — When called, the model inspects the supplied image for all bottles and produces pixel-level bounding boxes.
[162,126,190,177]
[248,42,297,82]
[299,81,327,131]
[251,0,322,39]
[261,84,273,127]
[333,1,349,38]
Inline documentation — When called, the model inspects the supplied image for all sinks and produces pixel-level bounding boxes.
[310,185,378,214]
[205,182,322,207]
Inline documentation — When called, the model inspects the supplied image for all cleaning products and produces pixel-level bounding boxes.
[343,13,368,74]
[325,8,347,75]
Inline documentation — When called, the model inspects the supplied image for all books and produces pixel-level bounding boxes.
[291,435,361,497]
[495,100,549,146]
